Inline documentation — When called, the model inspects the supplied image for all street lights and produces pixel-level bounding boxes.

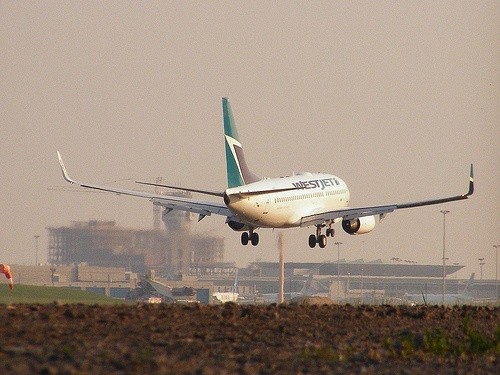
[334,241,343,305]
[493,244,500,301]
[440,210,450,306]
[477,257,485,280]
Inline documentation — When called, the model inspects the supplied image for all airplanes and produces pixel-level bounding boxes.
[54,95,475,249]
